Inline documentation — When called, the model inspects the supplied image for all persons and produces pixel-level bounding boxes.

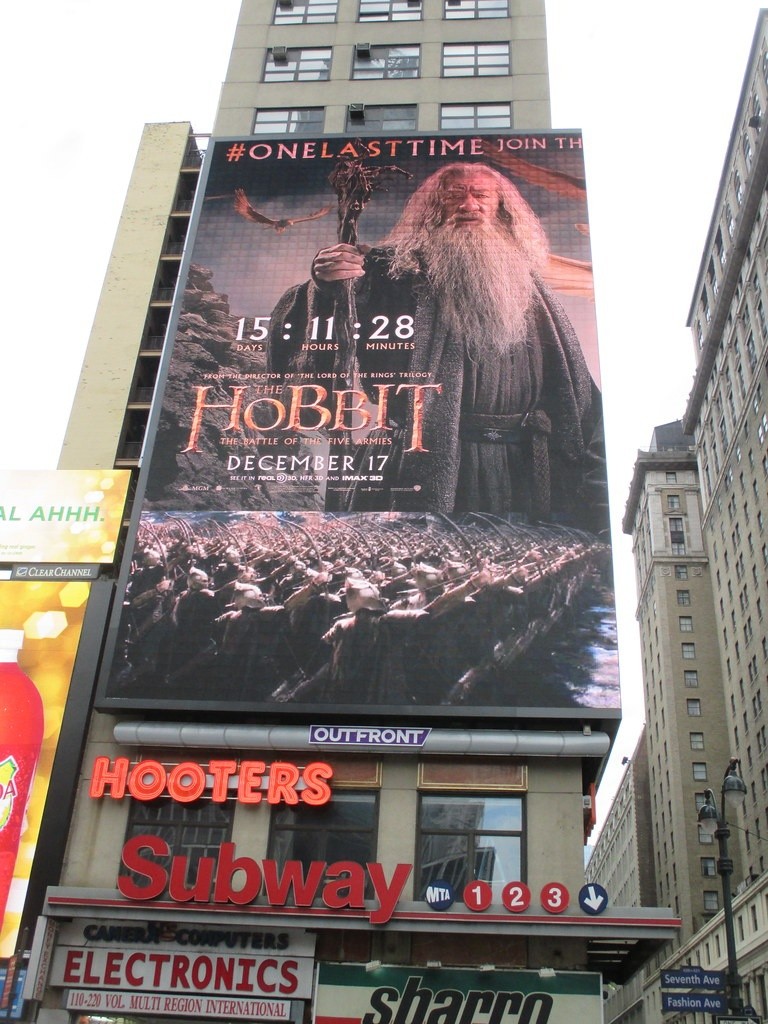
[264,160,609,532]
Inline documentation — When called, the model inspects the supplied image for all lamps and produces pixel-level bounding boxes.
[608,982,620,992]
[427,959,441,968]
[365,959,381,972]
[537,966,556,978]
[479,962,494,971]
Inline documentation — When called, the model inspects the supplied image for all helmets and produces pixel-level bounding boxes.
[138,528,593,611]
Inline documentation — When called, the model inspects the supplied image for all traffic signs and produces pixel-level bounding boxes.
[714,1013,762,1024]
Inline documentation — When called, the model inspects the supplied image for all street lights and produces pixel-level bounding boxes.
[698,757,747,1016]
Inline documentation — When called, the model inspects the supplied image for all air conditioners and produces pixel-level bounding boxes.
[272,46,287,60]
[279,0,293,5]
[348,103,365,118]
[356,43,371,57]
